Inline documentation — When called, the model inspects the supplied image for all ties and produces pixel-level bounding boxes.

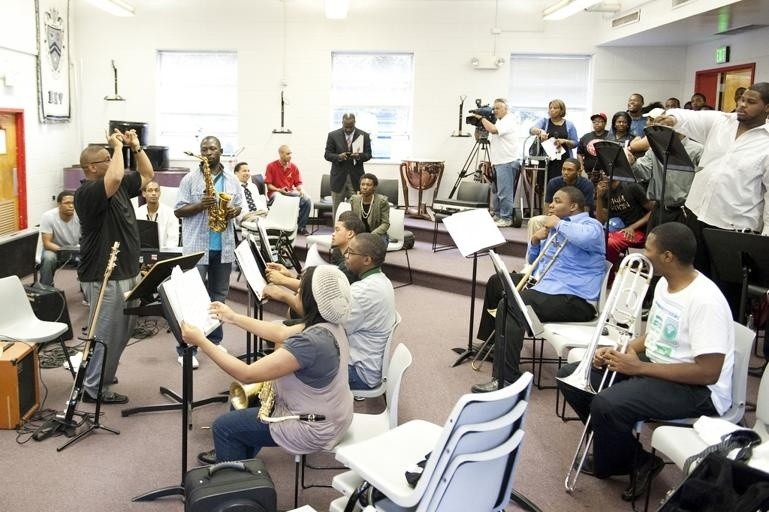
[242,183,257,211]
[346,134,352,150]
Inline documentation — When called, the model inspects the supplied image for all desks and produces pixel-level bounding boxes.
[1,341,41,429]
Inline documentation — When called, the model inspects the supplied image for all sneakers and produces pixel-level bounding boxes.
[217,344,227,353]
[495,217,513,228]
[178,355,199,368]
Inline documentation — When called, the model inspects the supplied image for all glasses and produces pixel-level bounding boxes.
[90,157,111,164]
[348,249,367,259]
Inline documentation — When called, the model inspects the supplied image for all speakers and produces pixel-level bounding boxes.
[23,283,73,345]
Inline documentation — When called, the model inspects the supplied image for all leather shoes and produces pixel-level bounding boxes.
[111,376,119,384]
[298,228,309,235]
[197,450,216,464]
[621,454,664,502]
[83,391,128,404]
[472,342,495,358]
[472,376,510,393]
[574,453,629,476]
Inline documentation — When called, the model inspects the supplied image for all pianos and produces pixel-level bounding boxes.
[58,244,188,264]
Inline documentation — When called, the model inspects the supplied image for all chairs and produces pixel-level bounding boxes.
[328,371,536,511]
[236,191,304,282]
[311,174,333,235]
[31,188,179,298]
[294,341,413,510]
[642,362,767,511]
[303,201,352,264]
[377,206,413,291]
[304,310,403,470]
[628,319,757,509]
[375,179,398,209]
[1,274,75,381]
[746,275,768,378]
[519,259,649,422]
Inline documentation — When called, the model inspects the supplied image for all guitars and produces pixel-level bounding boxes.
[65,242,122,421]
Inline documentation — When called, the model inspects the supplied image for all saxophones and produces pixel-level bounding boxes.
[184,151,231,233]
[229,381,325,424]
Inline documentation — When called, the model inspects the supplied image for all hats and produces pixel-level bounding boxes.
[641,108,664,119]
[603,217,625,232]
[311,264,353,323]
[590,113,606,123]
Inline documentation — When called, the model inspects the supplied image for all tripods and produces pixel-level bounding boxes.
[132,350,192,502]
[236,301,274,360]
[451,254,493,367]
[448,139,497,198]
[57,340,120,450]
[122,312,227,417]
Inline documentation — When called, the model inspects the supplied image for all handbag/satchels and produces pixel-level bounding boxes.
[529,118,547,156]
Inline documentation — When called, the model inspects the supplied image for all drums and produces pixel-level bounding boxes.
[403,158,445,189]
[87,120,171,171]
[523,156,550,166]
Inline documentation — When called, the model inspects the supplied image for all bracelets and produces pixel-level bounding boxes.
[132,147,144,154]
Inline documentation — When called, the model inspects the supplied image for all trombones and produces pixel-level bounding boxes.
[471,232,568,370]
[555,254,654,493]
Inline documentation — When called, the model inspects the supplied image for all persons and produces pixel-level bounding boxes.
[33,191,80,287]
[74,126,155,405]
[467,80,768,503]
[174,113,395,466]
[135,182,179,252]
